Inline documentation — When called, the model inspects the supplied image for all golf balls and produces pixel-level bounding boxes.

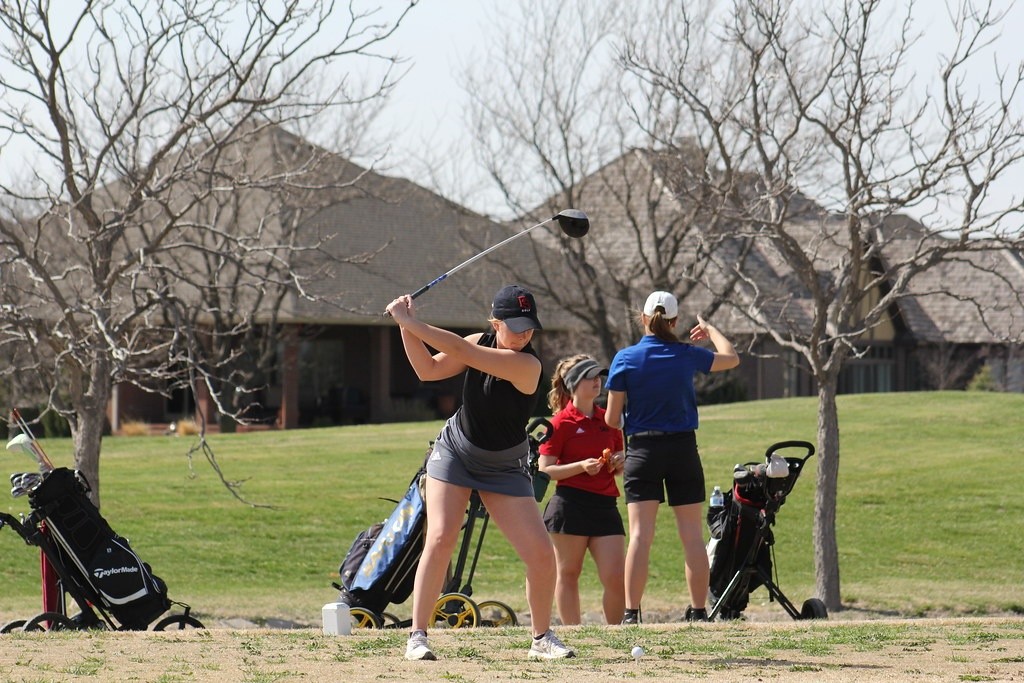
[630,646,644,657]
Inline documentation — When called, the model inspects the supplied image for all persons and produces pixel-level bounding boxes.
[386,284,575,663]
[603,291,740,627]
[537,352,628,625]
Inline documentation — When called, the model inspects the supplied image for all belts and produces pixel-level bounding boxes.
[634,429,674,435]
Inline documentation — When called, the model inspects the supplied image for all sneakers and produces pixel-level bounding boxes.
[405,630,437,661]
[528,630,576,659]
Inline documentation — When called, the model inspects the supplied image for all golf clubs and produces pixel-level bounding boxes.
[381,209,590,319]
[734,462,767,499]
[5,407,52,498]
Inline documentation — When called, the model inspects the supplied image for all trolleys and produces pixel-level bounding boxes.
[332,415,555,632]
[700,440,829,620]
[0,408,208,631]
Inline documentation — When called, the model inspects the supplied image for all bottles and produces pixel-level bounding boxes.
[709,485,723,507]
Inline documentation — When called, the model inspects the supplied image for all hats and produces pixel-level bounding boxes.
[642,291,678,319]
[492,285,543,333]
[563,360,609,393]
